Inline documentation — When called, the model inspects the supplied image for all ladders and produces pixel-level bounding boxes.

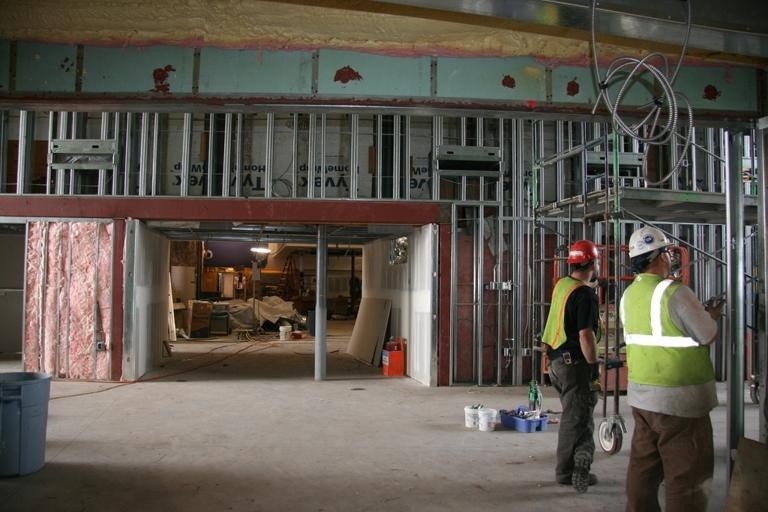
[277,254,298,291]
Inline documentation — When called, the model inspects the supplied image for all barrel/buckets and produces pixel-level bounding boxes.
[477,408,497,432]
[279,325,292,341]
[464,405,486,429]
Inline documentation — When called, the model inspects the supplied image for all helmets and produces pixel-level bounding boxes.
[626,224,676,260]
[565,238,599,265]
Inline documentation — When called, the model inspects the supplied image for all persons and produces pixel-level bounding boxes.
[540,239,603,494]
[617,226,728,511]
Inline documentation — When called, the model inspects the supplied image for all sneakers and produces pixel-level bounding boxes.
[557,472,598,486]
[570,445,594,494]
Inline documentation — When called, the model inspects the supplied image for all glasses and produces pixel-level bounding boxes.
[660,247,674,257]
